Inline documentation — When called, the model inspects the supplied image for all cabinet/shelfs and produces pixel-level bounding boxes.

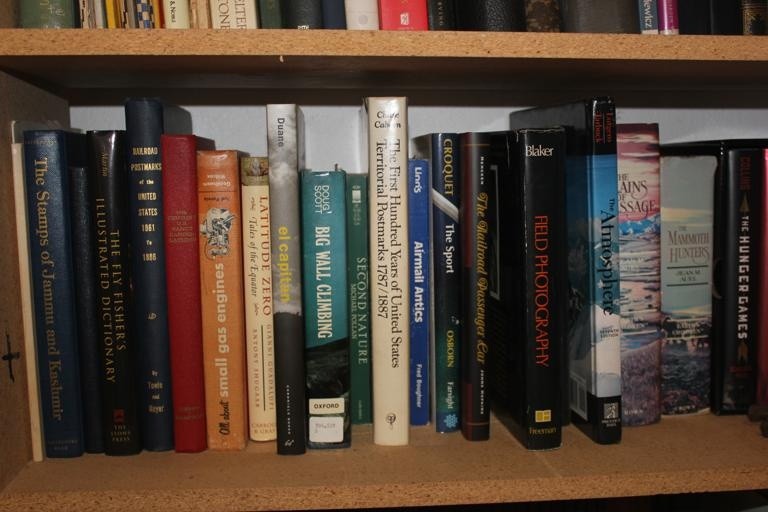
[0,0,768,512]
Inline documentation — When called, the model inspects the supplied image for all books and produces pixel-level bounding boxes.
[409,160,431,428]
[426,0,527,31]
[663,133,767,415]
[488,128,564,451]
[523,1,639,33]
[86,129,144,455]
[359,95,410,447]
[78,0,161,29]
[197,150,249,452]
[208,0,258,30]
[614,122,662,428]
[677,0,743,35]
[299,171,350,449]
[241,155,276,442]
[21,129,87,457]
[160,135,208,454]
[347,173,372,425]
[258,0,347,30]
[657,0,680,36]
[123,96,174,452]
[162,0,212,30]
[17,0,75,29]
[71,166,104,453]
[638,1,659,35]
[266,102,309,455]
[663,155,719,414]
[413,133,491,442]
[510,97,623,444]
[378,0,428,31]
